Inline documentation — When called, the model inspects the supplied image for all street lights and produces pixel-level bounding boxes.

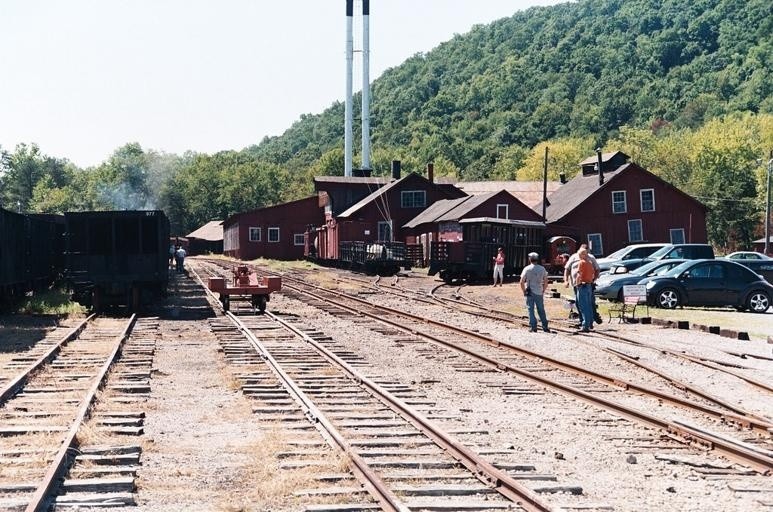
[755,158,772,254]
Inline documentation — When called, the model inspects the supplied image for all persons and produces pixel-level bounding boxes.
[576,248,595,333]
[520,252,550,333]
[169,242,187,274]
[563,243,603,327]
[492,247,505,287]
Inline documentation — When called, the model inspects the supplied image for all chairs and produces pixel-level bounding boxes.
[608,296,639,324]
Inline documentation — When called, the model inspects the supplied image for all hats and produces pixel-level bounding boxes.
[528,253,538,257]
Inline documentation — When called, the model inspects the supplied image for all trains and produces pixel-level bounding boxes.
[428,217,576,286]
[303,218,424,274]
[0,208,64,315]
[64,212,170,314]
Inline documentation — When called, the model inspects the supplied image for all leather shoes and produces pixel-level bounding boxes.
[575,318,602,332]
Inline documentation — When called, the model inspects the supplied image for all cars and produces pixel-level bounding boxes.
[594,242,773,313]
